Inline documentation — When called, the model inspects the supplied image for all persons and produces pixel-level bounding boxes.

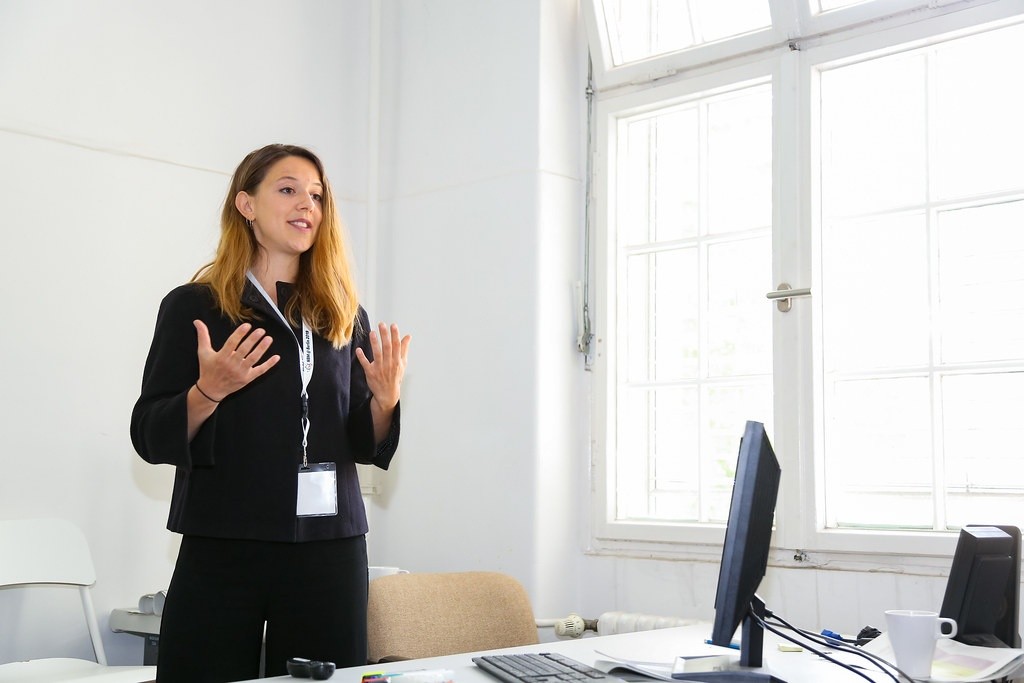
[130,144,414,683]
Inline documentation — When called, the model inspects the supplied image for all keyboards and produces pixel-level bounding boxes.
[471,652,627,683]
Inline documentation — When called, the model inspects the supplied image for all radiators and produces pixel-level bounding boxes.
[596,612,702,636]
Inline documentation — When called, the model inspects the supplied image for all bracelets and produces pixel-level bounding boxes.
[195,382,222,404]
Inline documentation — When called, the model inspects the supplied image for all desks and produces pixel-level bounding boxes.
[226,618,898,683]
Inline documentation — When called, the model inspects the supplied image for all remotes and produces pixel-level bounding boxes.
[285,658,336,681]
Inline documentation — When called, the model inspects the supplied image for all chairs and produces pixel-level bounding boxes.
[362,571,540,663]
[0,520,157,683]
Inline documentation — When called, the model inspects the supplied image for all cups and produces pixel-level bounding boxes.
[885,609,957,678]
[368,566,410,580]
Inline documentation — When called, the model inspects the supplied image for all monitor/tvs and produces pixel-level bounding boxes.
[672,419,782,683]
